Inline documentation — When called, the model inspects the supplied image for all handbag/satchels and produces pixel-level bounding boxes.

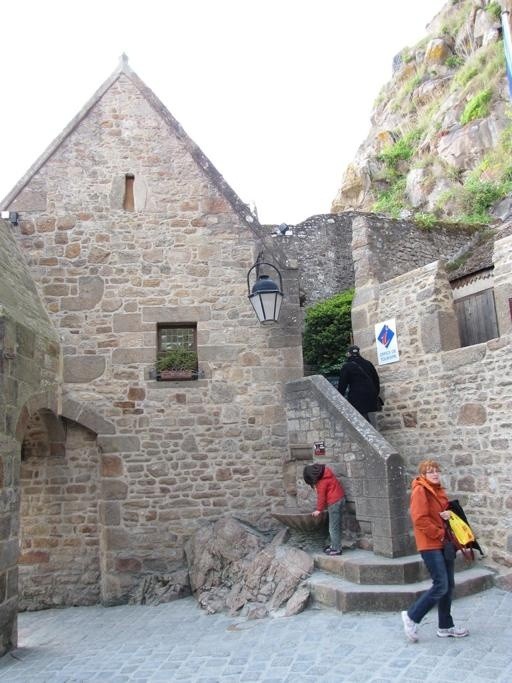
[376,397,384,411]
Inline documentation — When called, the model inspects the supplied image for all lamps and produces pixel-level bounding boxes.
[247,250,283,325]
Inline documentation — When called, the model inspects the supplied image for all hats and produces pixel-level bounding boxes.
[348,346,359,353]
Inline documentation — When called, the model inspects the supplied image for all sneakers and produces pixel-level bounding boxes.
[401,611,418,642]
[437,626,469,637]
[324,548,342,556]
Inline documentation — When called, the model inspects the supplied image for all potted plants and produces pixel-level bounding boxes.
[155,342,198,380]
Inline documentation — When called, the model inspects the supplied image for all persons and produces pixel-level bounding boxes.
[401,459,469,642]
[338,345,379,423]
[303,463,346,555]
